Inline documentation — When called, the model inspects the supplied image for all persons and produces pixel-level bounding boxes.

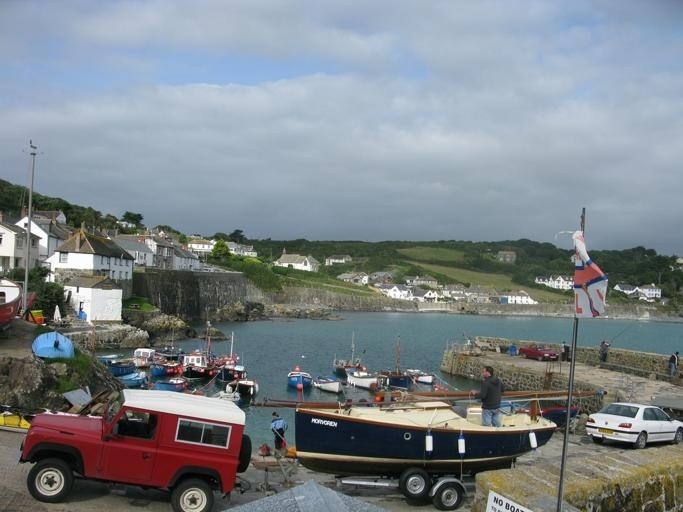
[600,340,611,361]
[560,340,566,361]
[668,351,679,376]
[472,366,505,426]
[270,411,288,448]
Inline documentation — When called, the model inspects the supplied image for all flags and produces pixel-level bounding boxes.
[569,230,609,317]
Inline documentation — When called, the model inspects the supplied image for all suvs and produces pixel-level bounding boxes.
[16,388,251,512]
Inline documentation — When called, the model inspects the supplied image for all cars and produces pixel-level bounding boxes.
[584,402,682,450]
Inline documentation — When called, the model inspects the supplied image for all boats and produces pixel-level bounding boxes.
[250,444,299,470]
[96,320,259,404]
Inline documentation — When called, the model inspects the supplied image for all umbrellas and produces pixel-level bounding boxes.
[53,306,61,323]
